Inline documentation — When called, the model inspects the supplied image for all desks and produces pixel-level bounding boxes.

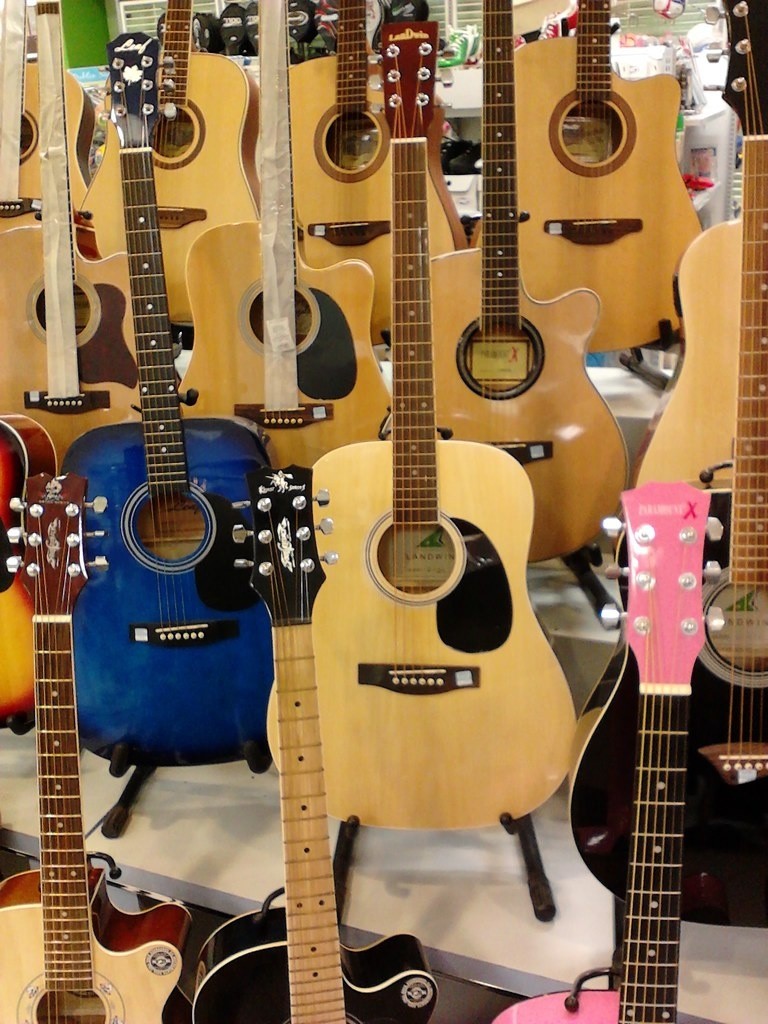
[0,726,768,1024]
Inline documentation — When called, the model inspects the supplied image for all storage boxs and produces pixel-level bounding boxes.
[608,46,677,81]
[443,174,478,213]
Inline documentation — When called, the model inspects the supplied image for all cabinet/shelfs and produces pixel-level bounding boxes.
[435,47,735,229]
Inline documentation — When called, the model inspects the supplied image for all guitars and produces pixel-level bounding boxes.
[0,413,59,729]
[56,31,277,769]
[288,0,468,362]
[1,473,193,1023]
[470,5,704,352]
[427,0,628,566]
[628,205,742,480]
[487,479,727,1023]
[174,0,394,467]
[1,0,144,476]
[189,462,440,1023]
[3,0,95,213]
[262,19,581,833]
[77,0,259,328]
[567,1,768,927]
